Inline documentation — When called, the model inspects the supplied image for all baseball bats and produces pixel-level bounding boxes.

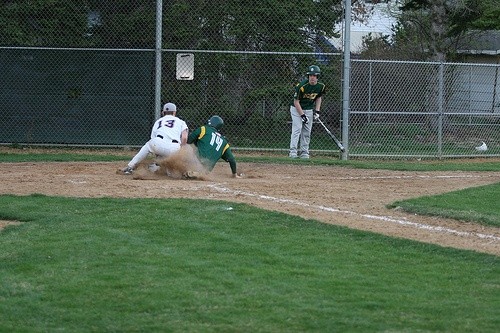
[315,114,346,153]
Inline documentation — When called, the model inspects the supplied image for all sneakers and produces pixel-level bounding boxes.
[184,171,193,178]
[300,152,310,158]
[125,167,134,175]
[288,153,297,159]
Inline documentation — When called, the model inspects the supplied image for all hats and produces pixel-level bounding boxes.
[163,103,176,111]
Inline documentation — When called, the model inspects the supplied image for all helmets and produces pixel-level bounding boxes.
[208,115,224,132]
[307,65,320,75]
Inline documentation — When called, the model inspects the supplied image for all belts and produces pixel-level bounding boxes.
[157,135,178,143]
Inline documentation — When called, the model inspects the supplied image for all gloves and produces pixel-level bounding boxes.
[300,114,309,124]
[313,110,321,119]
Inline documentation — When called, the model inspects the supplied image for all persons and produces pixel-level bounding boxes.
[123,102,189,174]
[289,65,325,159]
[187,116,241,178]
